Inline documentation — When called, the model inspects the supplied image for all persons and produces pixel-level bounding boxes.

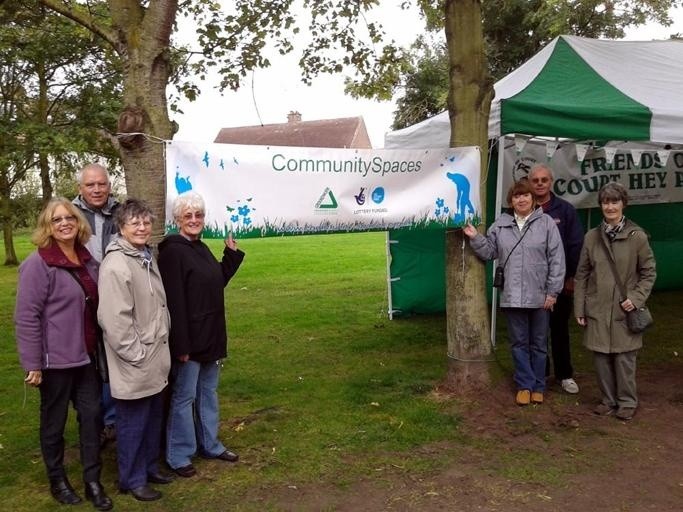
[13,197,113,511]
[527,162,584,394]
[460,178,567,405]
[70,163,122,448]
[574,181,657,421]
[97,198,173,502]
[156,189,245,477]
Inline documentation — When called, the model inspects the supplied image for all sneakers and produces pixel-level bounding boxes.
[593,401,615,417]
[560,377,580,394]
[530,390,545,404]
[614,405,638,420]
[102,422,118,442]
[515,387,531,406]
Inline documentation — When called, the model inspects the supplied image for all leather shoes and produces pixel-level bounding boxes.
[147,469,176,484]
[118,483,161,502]
[83,480,113,511]
[215,447,239,462]
[49,474,83,505]
[173,463,196,478]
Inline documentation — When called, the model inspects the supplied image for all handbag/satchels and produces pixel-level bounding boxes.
[492,265,505,289]
[627,305,655,335]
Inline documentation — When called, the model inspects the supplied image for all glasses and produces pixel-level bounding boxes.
[124,221,152,229]
[531,177,549,184]
[180,212,205,220]
